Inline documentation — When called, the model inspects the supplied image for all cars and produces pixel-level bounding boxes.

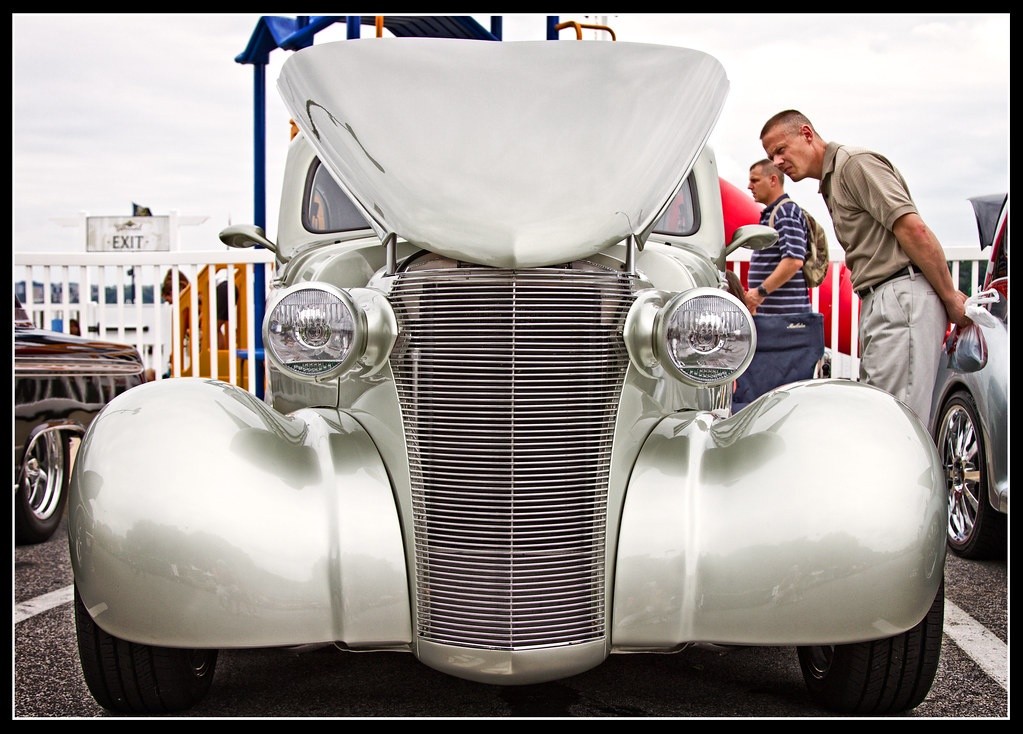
[70,38,949,719]
[930,188,1008,558]
[14,294,146,545]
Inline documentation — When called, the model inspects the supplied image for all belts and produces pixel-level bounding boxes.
[857,265,923,299]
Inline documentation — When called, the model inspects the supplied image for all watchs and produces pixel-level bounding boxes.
[758,285,767,297]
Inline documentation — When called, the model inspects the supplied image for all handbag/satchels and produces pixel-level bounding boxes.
[947,289,1000,372]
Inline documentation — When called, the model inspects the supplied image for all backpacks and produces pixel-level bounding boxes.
[769,198,829,287]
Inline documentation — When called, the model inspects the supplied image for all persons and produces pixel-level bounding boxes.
[759,109,973,430]
[161,268,226,371]
[70,319,81,336]
[745,158,812,316]
